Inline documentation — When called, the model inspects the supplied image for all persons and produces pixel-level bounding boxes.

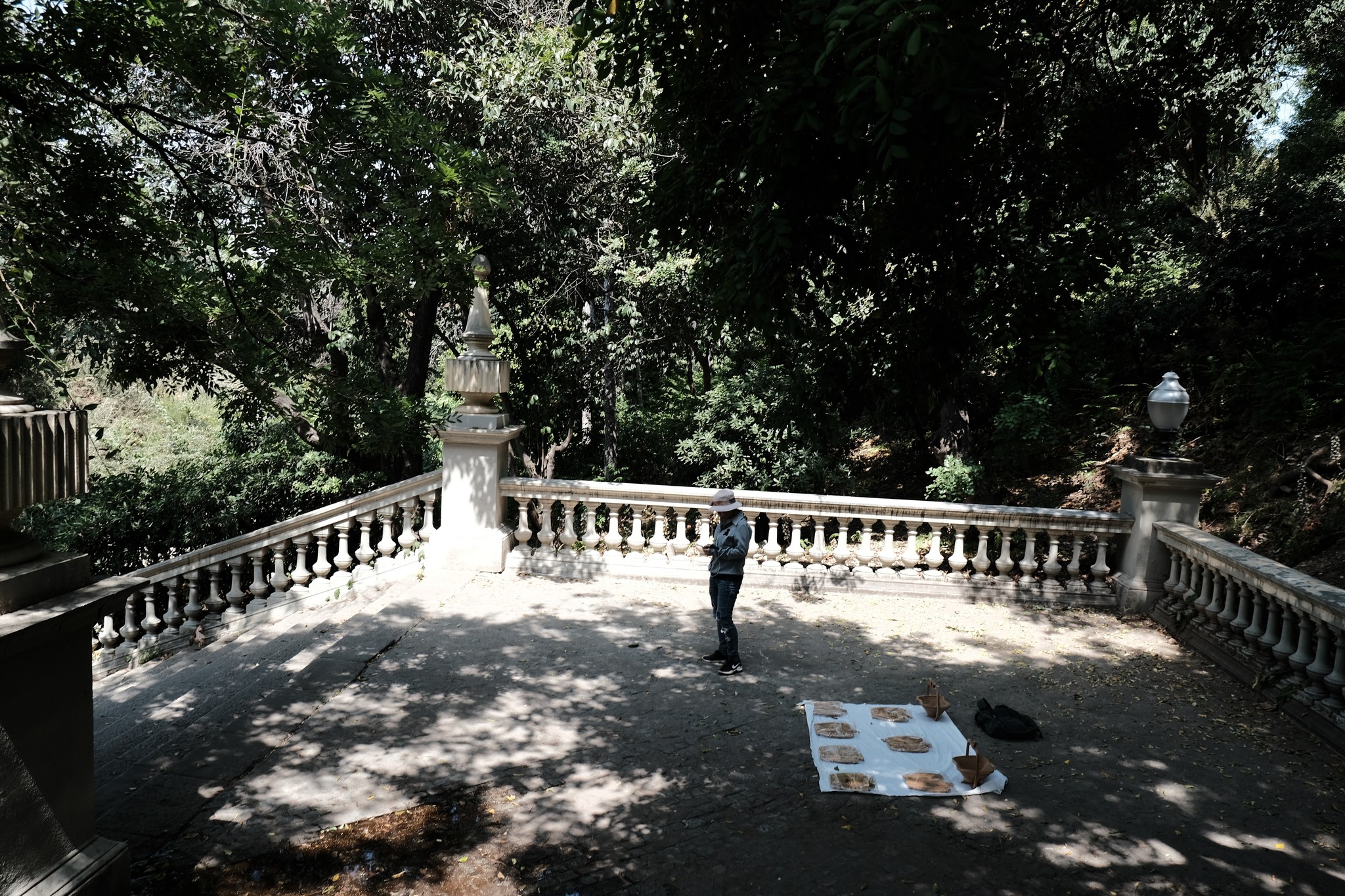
[700,488,752,675]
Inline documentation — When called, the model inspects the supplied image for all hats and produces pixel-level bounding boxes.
[708,487,742,511]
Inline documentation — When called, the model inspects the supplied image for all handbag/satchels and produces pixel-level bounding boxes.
[975,698,1042,743]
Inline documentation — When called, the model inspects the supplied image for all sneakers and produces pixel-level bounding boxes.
[718,658,743,676]
[702,649,727,663]
